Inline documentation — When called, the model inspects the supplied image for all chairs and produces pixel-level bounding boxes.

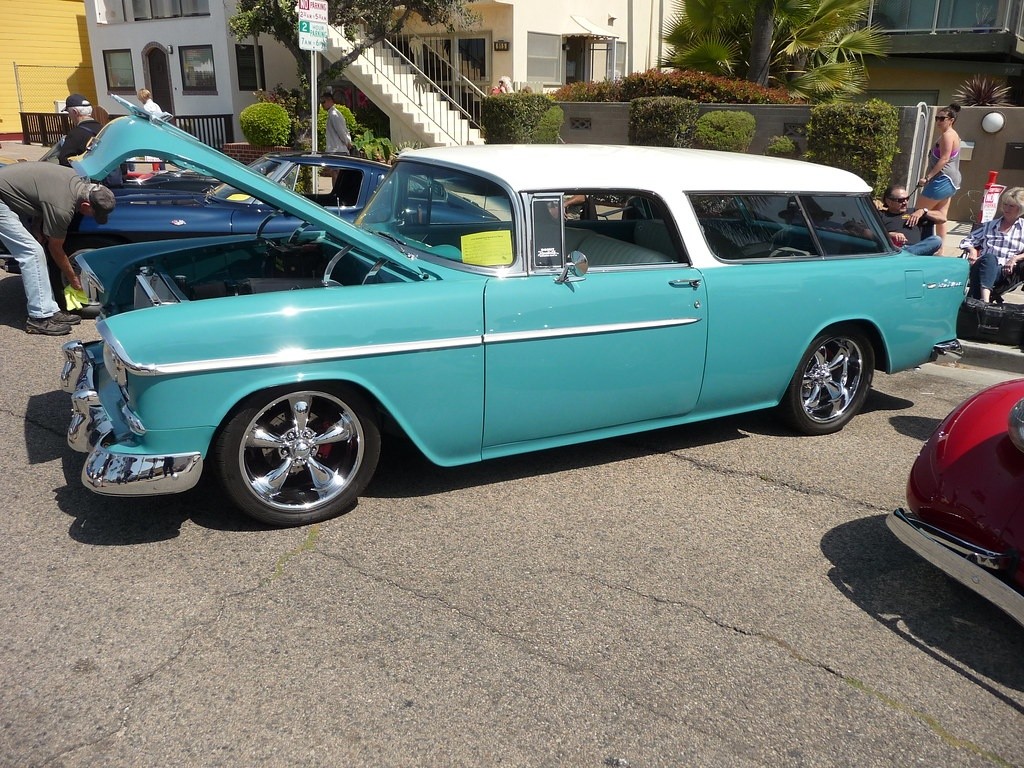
[540,226,587,261]
[960,239,1024,303]
[923,222,941,255]
[576,233,669,267]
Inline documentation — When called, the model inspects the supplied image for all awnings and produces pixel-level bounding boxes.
[563,16,619,40]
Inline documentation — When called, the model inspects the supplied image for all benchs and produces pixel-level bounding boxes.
[680,219,881,261]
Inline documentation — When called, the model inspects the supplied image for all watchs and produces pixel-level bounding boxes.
[923,208,928,215]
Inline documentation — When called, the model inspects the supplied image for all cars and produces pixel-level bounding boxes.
[886,377,1024,626]
[52,94,970,528]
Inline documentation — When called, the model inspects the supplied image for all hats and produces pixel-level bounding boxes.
[89,182,116,224]
[61,94,91,112]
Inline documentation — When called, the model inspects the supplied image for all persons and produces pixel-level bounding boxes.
[770,196,883,257]
[916,103,962,255]
[547,194,585,220]
[0,162,116,336]
[878,185,947,256]
[320,93,351,187]
[490,76,514,96]
[137,88,165,170]
[58,94,122,185]
[959,186,1024,303]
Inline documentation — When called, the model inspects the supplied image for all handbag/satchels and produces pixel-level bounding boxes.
[956,296,1024,345]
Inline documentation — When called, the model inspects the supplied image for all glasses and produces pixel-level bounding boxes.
[888,198,908,203]
[935,116,953,122]
[320,100,330,104]
[499,81,504,84]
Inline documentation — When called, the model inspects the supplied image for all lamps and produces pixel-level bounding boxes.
[981,110,1006,135]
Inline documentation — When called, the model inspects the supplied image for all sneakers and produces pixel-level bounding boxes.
[24,317,71,336]
[49,311,81,325]
